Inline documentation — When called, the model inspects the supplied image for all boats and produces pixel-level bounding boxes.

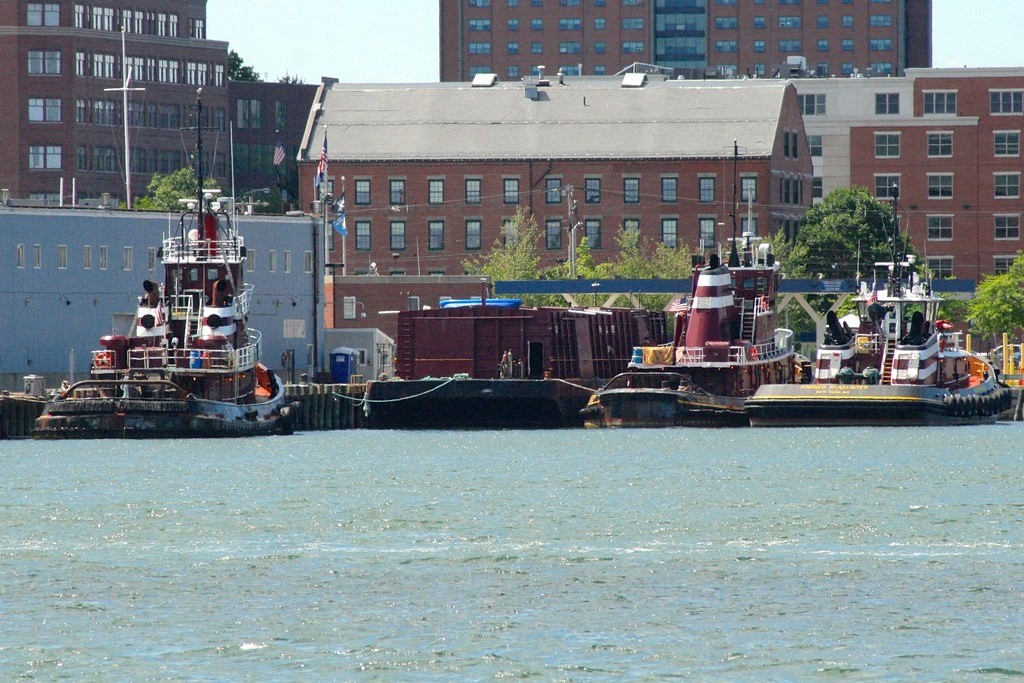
[578,200,813,428]
[33,122,304,440]
[742,182,1014,428]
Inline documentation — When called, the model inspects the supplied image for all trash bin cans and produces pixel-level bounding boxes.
[190,351,203,369]
[633,349,643,363]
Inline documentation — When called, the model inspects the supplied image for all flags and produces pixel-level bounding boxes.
[315,131,328,187]
[273,134,286,167]
[332,191,348,236]
[867,282,878,303]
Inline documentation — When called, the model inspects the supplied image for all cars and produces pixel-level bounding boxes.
[976,343,1024,372]
